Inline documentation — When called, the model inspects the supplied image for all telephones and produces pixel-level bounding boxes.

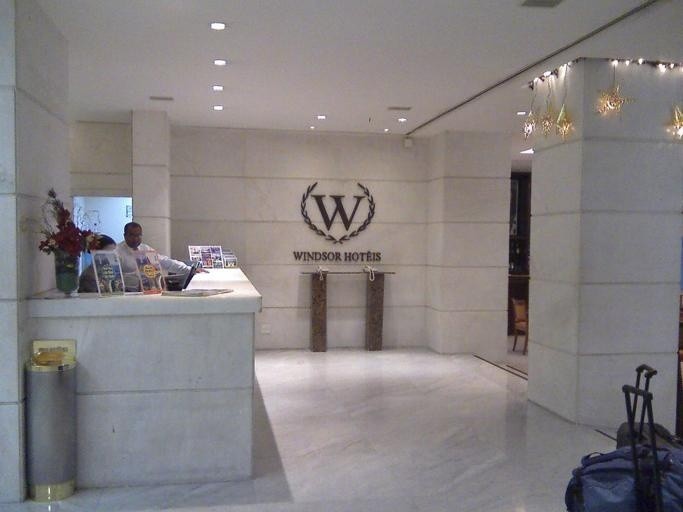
[363,264,377,273]
[314,264,329,272]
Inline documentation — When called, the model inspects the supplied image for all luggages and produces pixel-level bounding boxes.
[616,364,683,451]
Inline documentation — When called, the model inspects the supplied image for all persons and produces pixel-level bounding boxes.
[78,233,135,293]
[116,221,210,292]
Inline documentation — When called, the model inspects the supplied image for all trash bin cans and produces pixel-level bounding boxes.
[24,339,80,503]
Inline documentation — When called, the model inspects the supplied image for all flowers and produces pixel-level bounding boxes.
[20,186,104,256]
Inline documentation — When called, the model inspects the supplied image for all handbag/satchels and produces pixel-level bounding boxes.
[565,444,683,512]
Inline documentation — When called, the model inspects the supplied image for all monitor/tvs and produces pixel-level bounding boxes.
[182,261,200,288]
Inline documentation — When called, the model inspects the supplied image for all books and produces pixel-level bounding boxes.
[161,289,233,297]
[221,248,237,268]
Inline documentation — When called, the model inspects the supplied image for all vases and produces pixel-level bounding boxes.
[55,249,80,300]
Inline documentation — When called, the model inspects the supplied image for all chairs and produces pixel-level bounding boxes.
[511,297,528,355]
